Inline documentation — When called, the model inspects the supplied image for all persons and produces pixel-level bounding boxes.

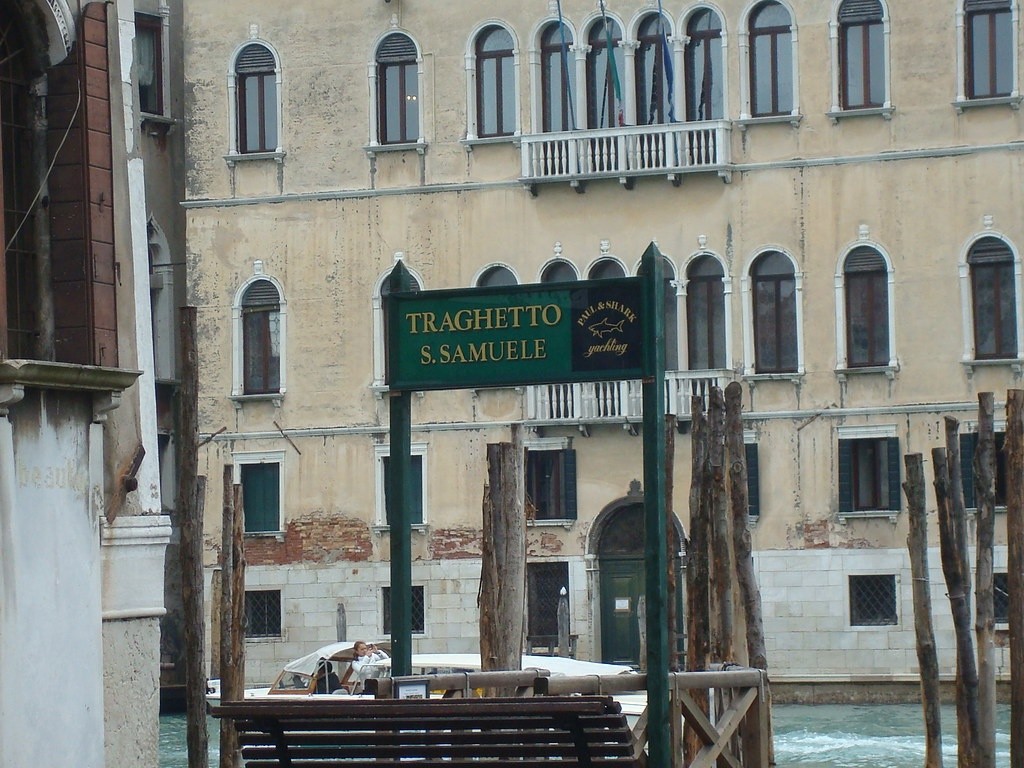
[311,662,344,695]
[352,640,388,696]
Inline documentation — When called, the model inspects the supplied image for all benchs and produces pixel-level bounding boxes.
[208,695,650,768]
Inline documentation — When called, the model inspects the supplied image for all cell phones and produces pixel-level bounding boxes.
[369,644,373,650]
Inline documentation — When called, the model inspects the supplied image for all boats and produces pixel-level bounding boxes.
[201,637,649,727]
